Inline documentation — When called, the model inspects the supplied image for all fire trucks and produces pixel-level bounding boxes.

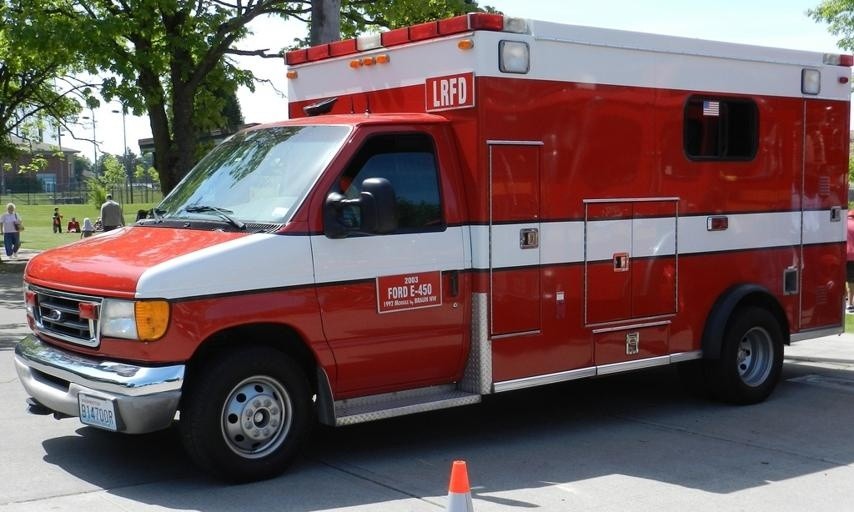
[15,13,854,484]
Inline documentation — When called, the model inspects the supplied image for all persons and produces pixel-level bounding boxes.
[846,209,854,313]
[53,194,125,239]
[0,203,24,259]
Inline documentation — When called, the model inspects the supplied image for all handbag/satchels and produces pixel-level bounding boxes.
[14,213,24,231]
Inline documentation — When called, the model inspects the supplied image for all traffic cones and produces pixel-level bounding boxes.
[445,460,475,512]
